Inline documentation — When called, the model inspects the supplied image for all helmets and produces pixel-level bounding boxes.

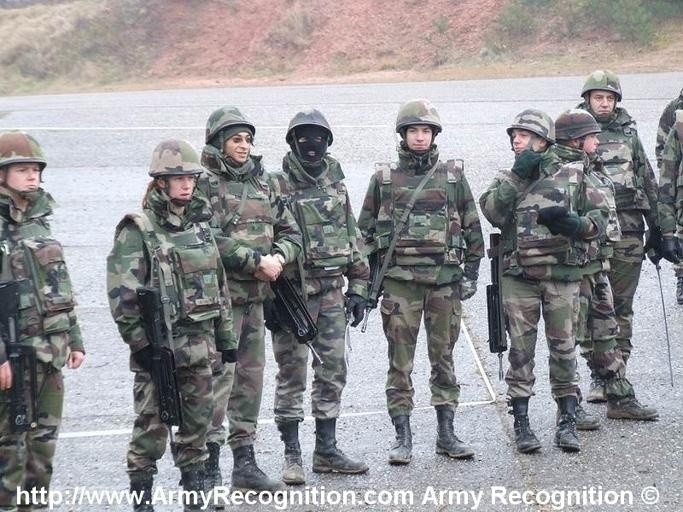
[0,129,47,171]
[286,109,333,147]
[554,109,601,141]
[506,108,555,145]
[580,69,622,101]
[205,106,255,144]
[148,139,204,176]
[396,98,442,133]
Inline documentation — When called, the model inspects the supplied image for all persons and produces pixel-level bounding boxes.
[268,107,370,485]
[657,107,683,275]
[655,91,683,304]
[105,139,238,512]
[0,128,85,509]
[573,68,662,403]
[191,106,303,511]
[555,106,659,430]
[356,100,484,463]
[498,109,608,450]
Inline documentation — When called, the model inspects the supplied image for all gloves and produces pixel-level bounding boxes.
[644,230,683,263]
[511,149,544,180]
[559,213,592,240]
[459,276,477,301]
[222,349,239,365]
[345,291,383,327]
[263,299,280,333]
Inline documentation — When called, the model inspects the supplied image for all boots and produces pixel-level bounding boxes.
[278,421,305,485]
[178,442,225,512]
[126,470,154,512]
[435,409,474,459]
[387,415,412,464]
[312,417,369,474]
[507,396,542,453]
[229,444,290,498]
[553,392,600,452]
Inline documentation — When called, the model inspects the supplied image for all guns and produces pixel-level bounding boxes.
[1,281,39,431]
[137,286,183,426]
[486,234,507,380]
[271,274,325,367]
[361,256,384,333]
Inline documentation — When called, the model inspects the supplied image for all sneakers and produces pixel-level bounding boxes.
[587,375,608,402]
[606,393,659,420]
